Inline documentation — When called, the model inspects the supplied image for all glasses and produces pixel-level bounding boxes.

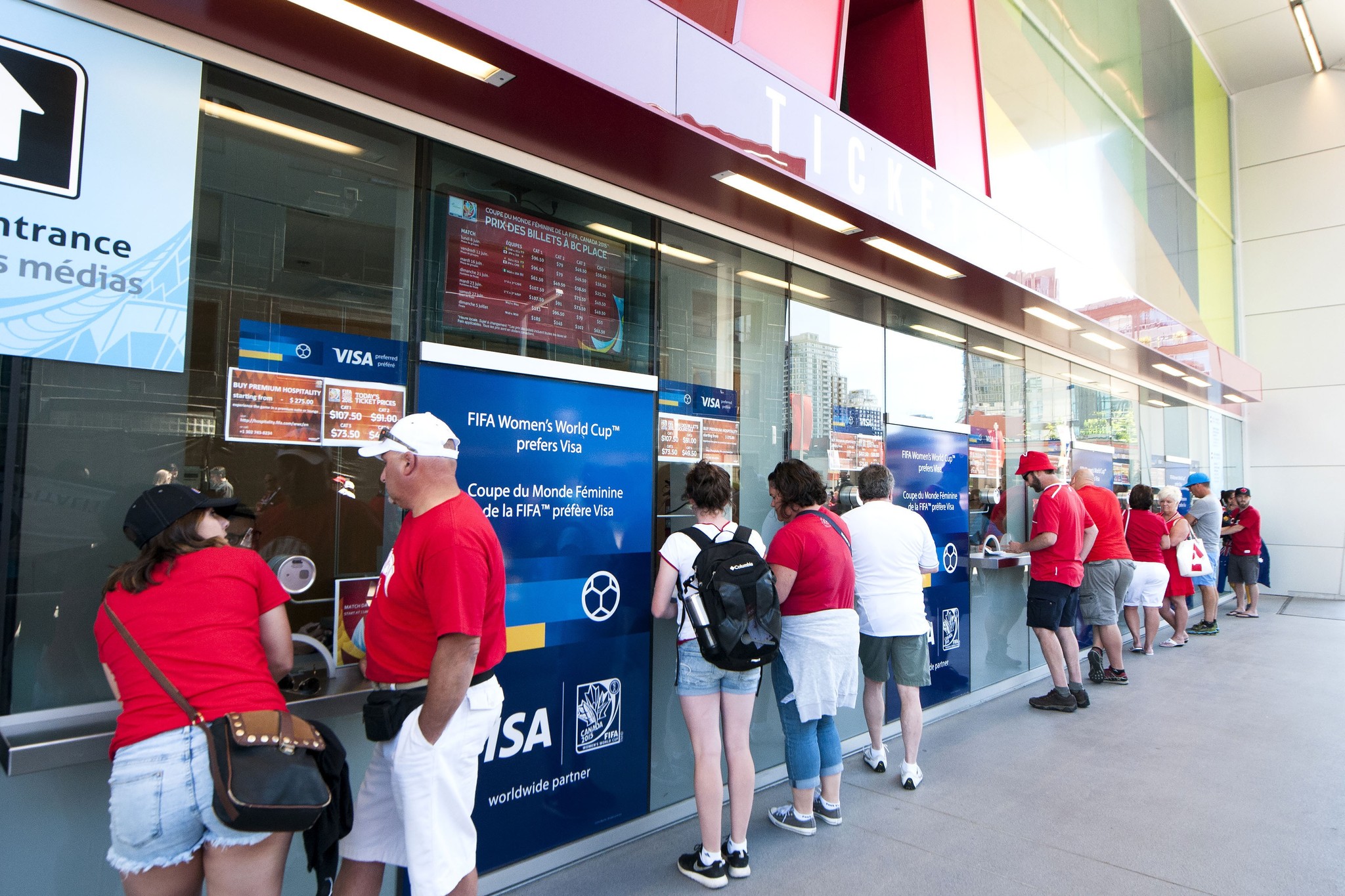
[1187,485,1193,489]
[378,427,419,455]
[1020,471,1032,481]
[276,438,331,460]
[225,530,261,545]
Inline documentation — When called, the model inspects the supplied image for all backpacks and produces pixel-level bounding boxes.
[672,525,781,671]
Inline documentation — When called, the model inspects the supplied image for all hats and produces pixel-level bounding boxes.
[1014,451,1058,474]
[123,484,244,550]
[1234,487,1250,498]
[357,411,460,460]
[1182,472,1210,487]
[274,426,336,466]
[331,475,355,490]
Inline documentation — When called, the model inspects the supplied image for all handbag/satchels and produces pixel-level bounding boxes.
[202,709,333,833]
[1175,539,1214,577]
[363,669,495,742]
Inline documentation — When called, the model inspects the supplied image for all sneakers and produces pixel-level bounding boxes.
[1028,682,1090,713]
[768,797,842,836]
[863,747,888,773]
[1088,648,1105,683]
[985,634,1022,667]
[900,763,924,790]
[676,842,750,889]
[1186,619,1220,636]
[1102,666,1129,685]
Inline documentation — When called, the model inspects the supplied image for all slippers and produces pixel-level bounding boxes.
[1128,644,1154,655]
[1160,636,1189,648]
[1227,610,1260,618]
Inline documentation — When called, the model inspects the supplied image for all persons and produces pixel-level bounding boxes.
[91,482,300,896]
[981,475,1016,547]
[1219,488,1260,613]
[1183,470,1222,637]
[15,435,384,710]
[1152,485,1196,647]
[1117,483,1173,656]
[970,543,992,553]
[981,481,1041,670]
[1067,467,1139,686]
[916,449,970,534]
[650,457,772,891]
[968,488,989,594]
[762,456,860,837]
[833,462,941,791]
[1217,487,1263,619]
[327,407,510,896]
[1000,449,1099,714]
